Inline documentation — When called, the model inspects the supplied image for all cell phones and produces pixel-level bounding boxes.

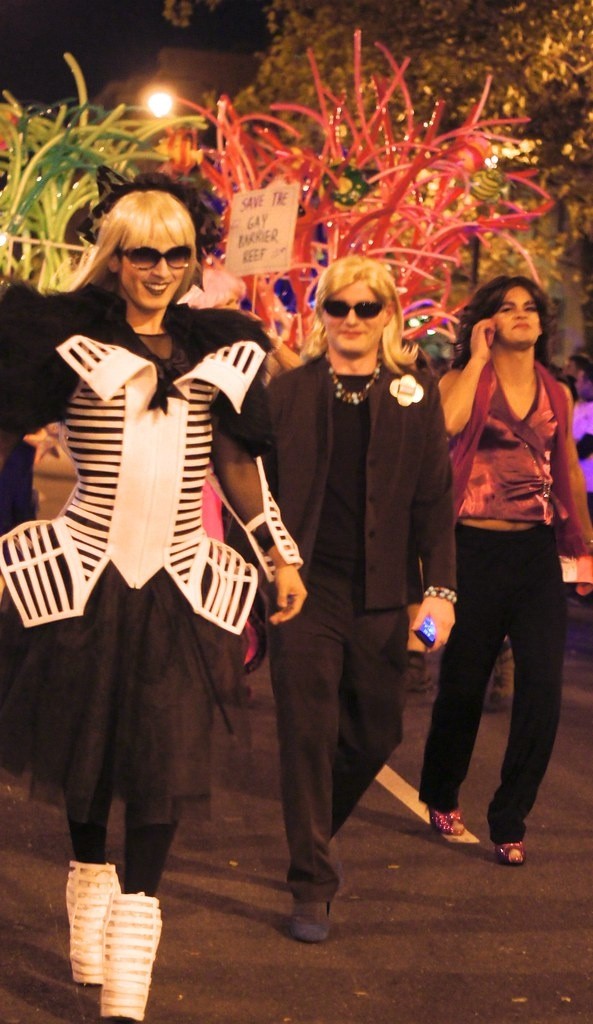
[414,613,437,647]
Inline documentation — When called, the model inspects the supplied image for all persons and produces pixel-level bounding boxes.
[0,162,308,1024]
[417,355,593,603]
[224,253,460,942]
[419,273,593,865]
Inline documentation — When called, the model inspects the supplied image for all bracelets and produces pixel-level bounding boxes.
[424,586,458,603]
[268,337,283,355]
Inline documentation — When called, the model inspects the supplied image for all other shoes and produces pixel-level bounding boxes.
[102,892,163,1023]
[485,664,517,711]
[406,649,439,693]
[66,862,122,983]
[290,899,332,942]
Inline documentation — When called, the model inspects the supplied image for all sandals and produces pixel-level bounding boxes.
[428,803,466,836]
[495,840,527,865]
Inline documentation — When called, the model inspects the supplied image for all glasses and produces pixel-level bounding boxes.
[118,245,194,270]
[324,298,383,319]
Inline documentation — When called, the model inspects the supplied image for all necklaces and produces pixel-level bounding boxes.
[324,345,383,405]
